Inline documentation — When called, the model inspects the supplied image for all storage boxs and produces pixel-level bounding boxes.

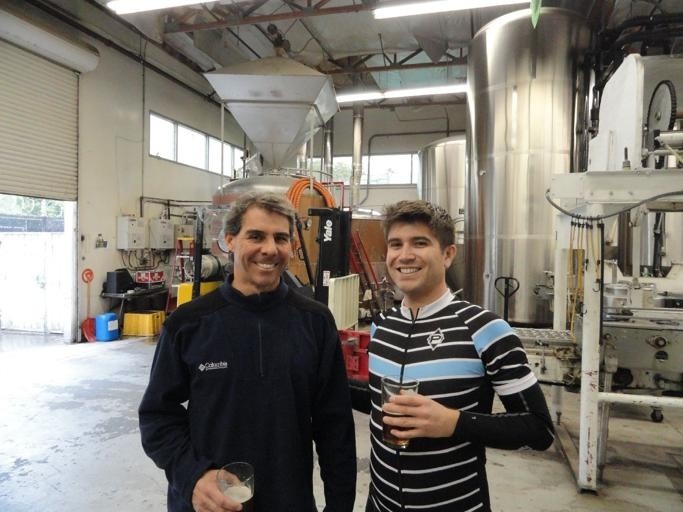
[122,309,166,337]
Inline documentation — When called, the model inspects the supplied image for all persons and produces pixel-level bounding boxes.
[346,198,555,512]
[138,194,356,512]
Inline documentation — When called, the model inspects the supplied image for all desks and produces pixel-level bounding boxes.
[100,287,168,330]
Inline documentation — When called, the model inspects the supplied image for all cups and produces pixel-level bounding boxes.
[380,375,420,450]
[216,461,255,512]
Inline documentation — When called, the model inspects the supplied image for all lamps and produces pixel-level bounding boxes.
[370,0,534,20]
[335,83,468,103]
